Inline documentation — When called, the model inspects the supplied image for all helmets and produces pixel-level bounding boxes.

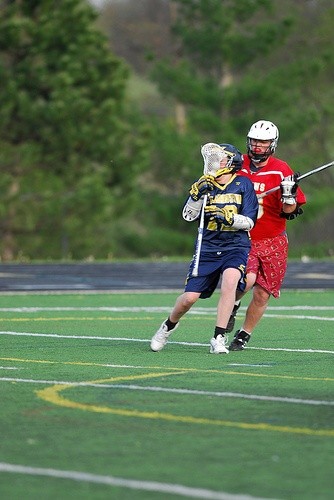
[216,144,244,178]
[247,120,279,163]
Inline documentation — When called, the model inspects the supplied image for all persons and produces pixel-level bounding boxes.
[222,120,306,351]
[150,143,259,354]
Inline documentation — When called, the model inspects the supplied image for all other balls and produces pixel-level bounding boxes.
[212,162,219,170]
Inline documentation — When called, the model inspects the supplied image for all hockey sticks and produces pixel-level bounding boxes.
[191,142,228,277]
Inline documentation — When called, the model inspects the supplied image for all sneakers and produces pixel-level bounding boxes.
[229,329,251,351]
[226,301,241,333]
[150,319,180,351]
[210,334,228,353]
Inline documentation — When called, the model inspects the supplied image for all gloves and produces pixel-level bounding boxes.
[189,175,215,201]
[280,172,300,205]
[204,206,234,227]
